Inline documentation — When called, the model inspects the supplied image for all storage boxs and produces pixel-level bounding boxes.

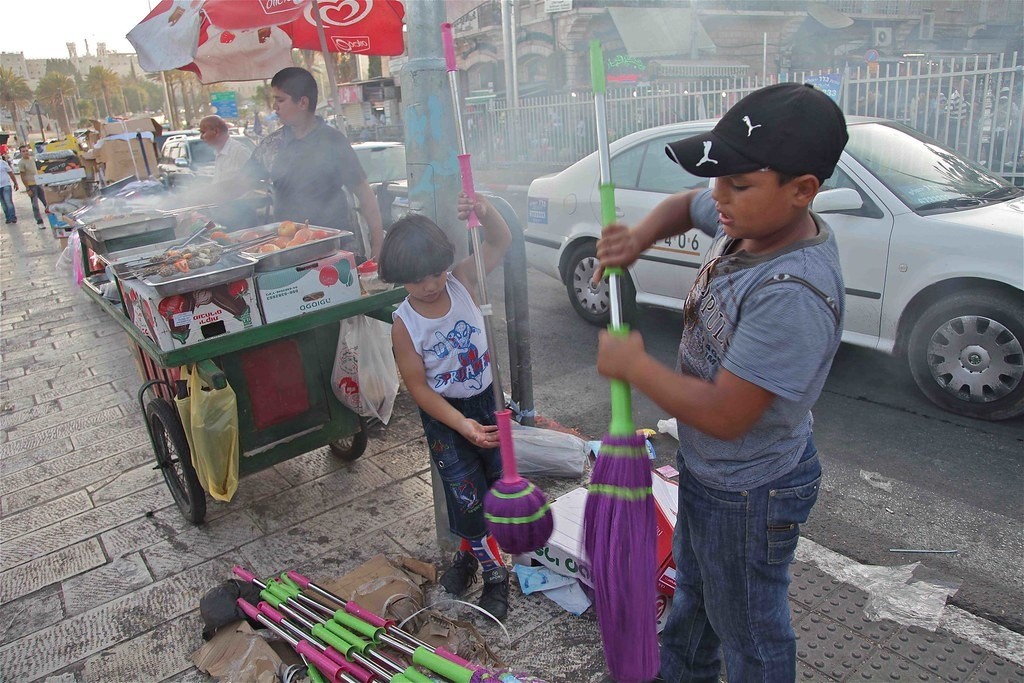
[253,250,361,325]
[85,118,163,149]
[34,134,96,206]
[350,141,407,183]
[511,470,679,632]
[47,213,74,237]
[117,278,264,352]
[589,440,656,461]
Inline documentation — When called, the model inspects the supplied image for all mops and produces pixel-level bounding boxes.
[228,561,542,683]
[576,37,659,680]
[439,17,554,554]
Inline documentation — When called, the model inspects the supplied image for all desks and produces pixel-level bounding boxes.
[370,180,408,231]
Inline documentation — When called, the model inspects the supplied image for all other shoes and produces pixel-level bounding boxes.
[38,222,46,230]
[5,219,16,224]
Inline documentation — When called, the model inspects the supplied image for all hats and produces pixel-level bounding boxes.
[664,82,851,180]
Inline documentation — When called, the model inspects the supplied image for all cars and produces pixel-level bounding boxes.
[523,115,1024,422]
[351,142,495,233]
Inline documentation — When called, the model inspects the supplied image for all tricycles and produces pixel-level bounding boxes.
[77,203,411,523]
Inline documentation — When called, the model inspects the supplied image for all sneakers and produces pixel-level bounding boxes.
[440,549,478,597]
[478,566,510,622]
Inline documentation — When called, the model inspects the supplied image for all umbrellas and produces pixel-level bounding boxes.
[126,0,405,138]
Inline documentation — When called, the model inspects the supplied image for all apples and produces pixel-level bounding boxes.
[211,220,330,253]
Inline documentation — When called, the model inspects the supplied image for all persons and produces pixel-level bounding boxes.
[0,157,18,225]
[19,145,50,230]
[377,189,514,622]
[844,66,1024,169]
[468,108,585,163]
[156,67,385,272]
[596,83,849,683]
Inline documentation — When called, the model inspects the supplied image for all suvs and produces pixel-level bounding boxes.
[158,135,258,194]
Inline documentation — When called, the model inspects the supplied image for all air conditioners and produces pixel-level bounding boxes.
[919,13,935,38]
[874,27,892,47]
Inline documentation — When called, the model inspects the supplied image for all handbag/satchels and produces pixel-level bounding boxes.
[173,360,240,503]
[332,315,400,428]
